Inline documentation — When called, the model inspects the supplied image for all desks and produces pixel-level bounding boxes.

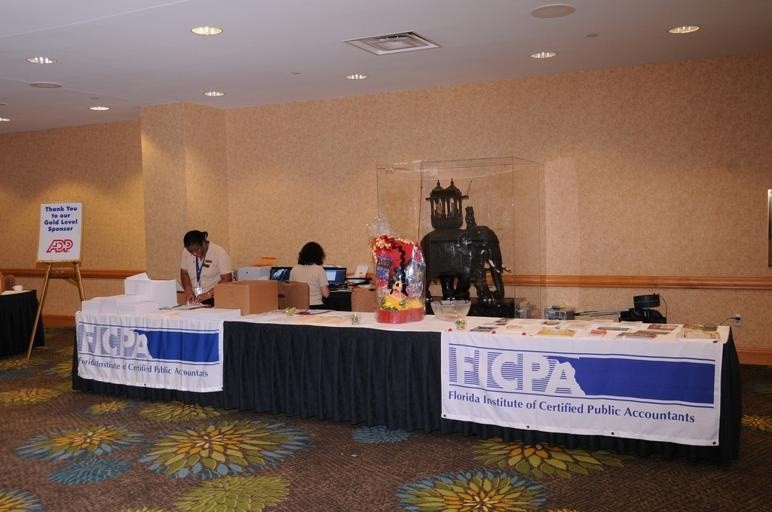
[71,294,745,470]
[1,285,49,361]
[321,283,380,314]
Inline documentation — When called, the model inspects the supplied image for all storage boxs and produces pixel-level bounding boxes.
[212,277,280,318]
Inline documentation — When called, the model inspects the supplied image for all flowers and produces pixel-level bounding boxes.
[368,234,424,308]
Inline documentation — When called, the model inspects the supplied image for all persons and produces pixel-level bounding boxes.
[289,242,332,308]
[179,230,233,306]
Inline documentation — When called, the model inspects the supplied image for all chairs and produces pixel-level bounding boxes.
[277,279,313,310]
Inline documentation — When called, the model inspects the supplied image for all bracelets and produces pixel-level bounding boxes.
[208,291,212,299]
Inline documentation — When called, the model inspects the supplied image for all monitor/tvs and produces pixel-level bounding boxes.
[270,266,290,279]
[238,266,270,280]
[322,266,346,286]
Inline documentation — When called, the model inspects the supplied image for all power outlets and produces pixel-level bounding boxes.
[733,313,744,327]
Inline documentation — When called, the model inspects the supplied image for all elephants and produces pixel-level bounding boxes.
[420,226,514,302]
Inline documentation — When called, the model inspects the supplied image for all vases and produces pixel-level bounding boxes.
[376,306,426,325]
